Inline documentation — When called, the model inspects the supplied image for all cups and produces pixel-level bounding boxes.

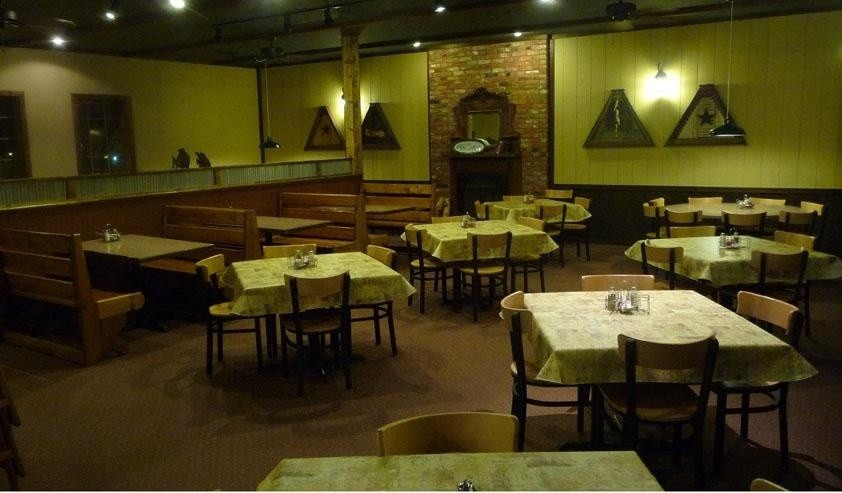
[109,233,117,241]
[639,294,651,314]
[737,199,744,208]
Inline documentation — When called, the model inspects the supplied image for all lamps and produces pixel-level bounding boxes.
[709,1,746,137]
[259,59,282,149]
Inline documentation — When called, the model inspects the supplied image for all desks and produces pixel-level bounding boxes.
[520,288,788,449]
[230,251,402,381]
[257,214,334,242]
[258,451,663,491]
[636,235,836,267]
[365,205,417,233]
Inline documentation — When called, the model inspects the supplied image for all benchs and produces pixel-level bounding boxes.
[273,190,367,252]
[138,203,263,289]
[0,228,145,367]
[360,182,438,233]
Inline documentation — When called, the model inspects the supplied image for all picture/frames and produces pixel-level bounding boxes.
[304,106,345,151]
[663,84,748,146]
[361,102,402,149]
[582,89,655,147]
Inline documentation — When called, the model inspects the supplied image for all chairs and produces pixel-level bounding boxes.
[350,245,400,355]
[197,254,270,376]
[598,330,720,476]
[750,248,810,335]
[405,190,825,323]
[711,290,803,471]
[262,244,318,258]
[498,291,605,450]
[748,479,784,490]
[280,269,353,394]
[375,412,517,455]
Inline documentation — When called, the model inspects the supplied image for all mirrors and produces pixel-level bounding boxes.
[447,88,522,153]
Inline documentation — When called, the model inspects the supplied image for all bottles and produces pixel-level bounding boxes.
[744,193,748,203]
[104,229,110,242]
[733,231,741,246]
[294,249,305,265]
[306,251,316,267]
[460,215,476,227]
[628,287,638,308]
[719,232,727,249]
[606,286,617,313]
[523,193,536,204]
